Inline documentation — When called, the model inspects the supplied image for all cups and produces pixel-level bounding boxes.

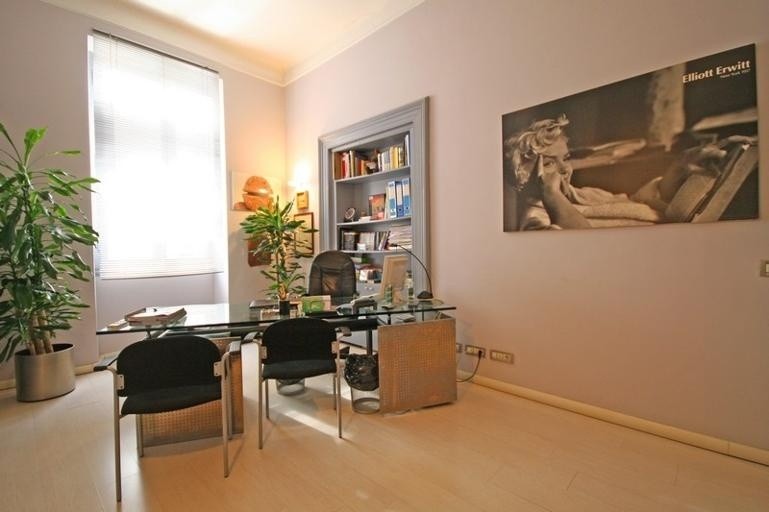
[280,301,290,315]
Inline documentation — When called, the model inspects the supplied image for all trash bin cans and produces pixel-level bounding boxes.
[346,353,381,415]
[276,378,305,396]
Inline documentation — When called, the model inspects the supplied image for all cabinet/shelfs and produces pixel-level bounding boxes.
[331,122,414,294]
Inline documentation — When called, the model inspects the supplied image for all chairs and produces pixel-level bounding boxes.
[307,250,356,298]
[94,336,241,502]
[243,317,344,448]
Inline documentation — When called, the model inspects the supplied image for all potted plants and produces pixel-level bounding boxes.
[0,122,99,403]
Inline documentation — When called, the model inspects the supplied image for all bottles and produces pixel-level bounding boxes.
[404,271,415,303]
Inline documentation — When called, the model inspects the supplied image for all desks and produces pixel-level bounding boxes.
[96,292,458,417]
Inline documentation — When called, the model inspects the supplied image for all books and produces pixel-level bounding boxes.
[332,135,411,180]
[336,224,413,250]
[124,306,186,323]
[351,253,382,284]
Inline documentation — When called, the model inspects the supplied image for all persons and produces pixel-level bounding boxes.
[505,114,727,229]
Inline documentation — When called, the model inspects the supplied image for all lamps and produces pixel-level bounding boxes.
[379,239,433,299]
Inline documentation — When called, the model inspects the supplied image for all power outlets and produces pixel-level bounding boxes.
[455,343,463,353]
[489,350,514,364]
[465,345,486,358]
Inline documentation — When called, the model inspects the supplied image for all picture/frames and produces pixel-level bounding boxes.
[292,211,314,257]
